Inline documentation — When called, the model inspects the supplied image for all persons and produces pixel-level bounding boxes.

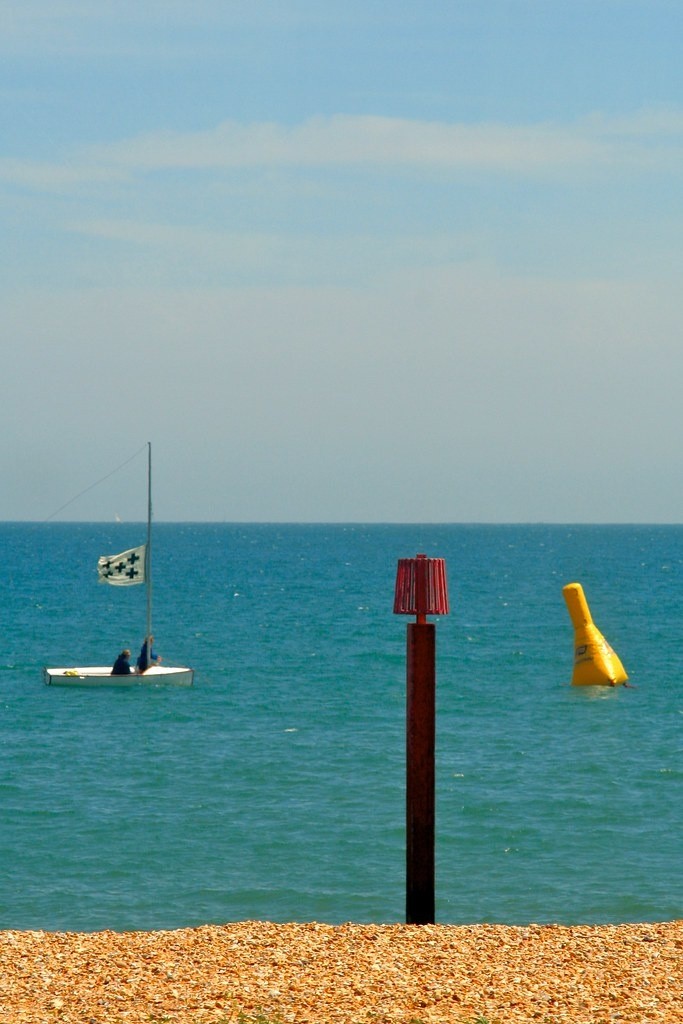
[112,649,135,675]
[137,635,162,674]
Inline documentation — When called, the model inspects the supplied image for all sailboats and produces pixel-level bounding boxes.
[43,439,195,688]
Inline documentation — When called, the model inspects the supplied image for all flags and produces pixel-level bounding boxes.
[97,544,147,585]
